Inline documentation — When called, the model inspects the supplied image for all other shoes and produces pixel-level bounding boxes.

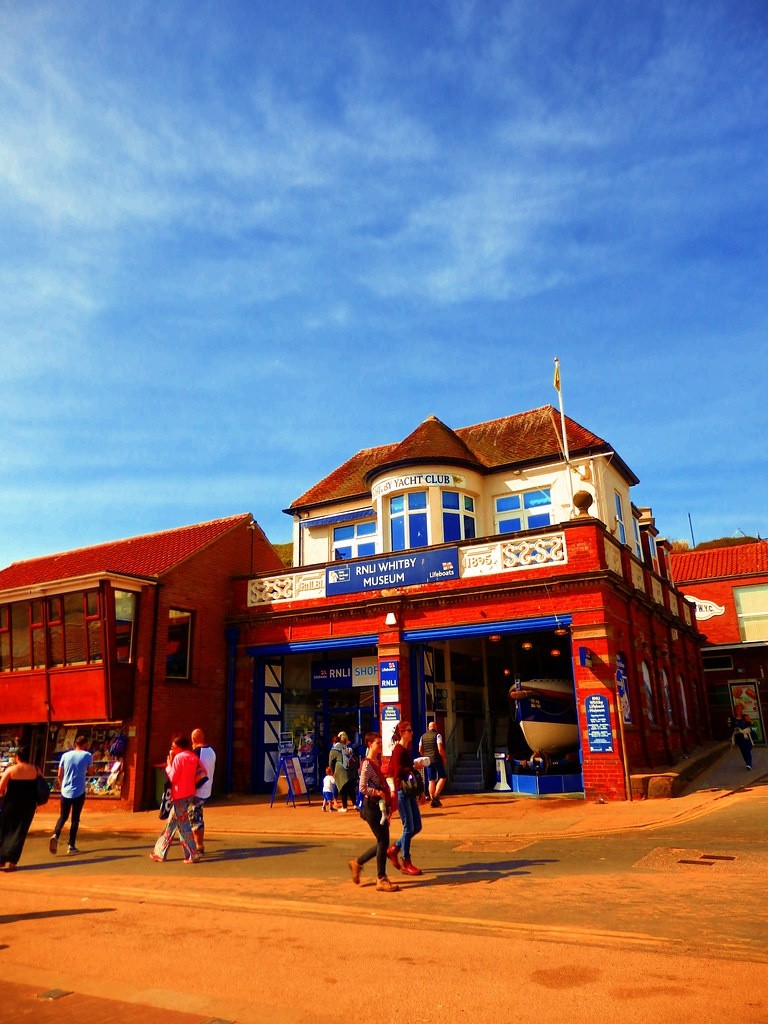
[338,807,347,812]
[376,875,398,891]
[430,800,438,807]
[322,807,326,812]
[67,847,78,853]
[349,858,364,885]
[49,834,58,854]
[354,805,357,809]
[746,765,752,770]
[197,849,205,857]
[329,808,337,812]
[149,853,163,861]
[183,855,200,863]
[432,796,442,807]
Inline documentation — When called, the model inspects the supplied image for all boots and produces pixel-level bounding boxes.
[386,843,402,869]
[401,854,423,876]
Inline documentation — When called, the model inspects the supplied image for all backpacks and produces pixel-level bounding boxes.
[331,746,355,769]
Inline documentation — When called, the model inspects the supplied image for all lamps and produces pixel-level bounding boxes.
[550,648,561,657]
[490,635,501,641]
[521,640,532,650]
[554,629,569,636]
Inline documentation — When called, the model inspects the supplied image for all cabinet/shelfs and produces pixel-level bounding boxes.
[44,760,112,779]
[278,745,317,792]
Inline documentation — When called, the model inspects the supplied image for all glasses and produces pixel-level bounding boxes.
[400,729,412,733]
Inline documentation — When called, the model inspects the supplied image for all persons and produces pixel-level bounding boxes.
[381,720,426,876]
[49,735,95,854]
[328,732,360,813]
[728,703,755,770]
[148,736,210,864]
[418,722,450,809]
[320,767,337,812]
[169,727,217,855]
[346,731,401,891]
[0,747,48,871]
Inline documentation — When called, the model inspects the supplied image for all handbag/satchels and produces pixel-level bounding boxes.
[107,733,127,786]
[749,729,758,741]
[34,764,50,805]
